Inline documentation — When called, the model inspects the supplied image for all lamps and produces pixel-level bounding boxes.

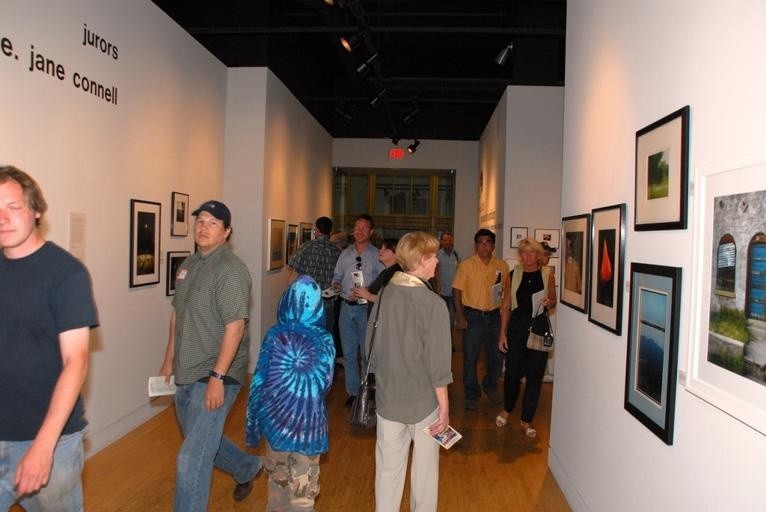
[323,0,423,155]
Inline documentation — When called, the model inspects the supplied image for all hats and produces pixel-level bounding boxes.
[538,240,557,254]
[191,200,231,224]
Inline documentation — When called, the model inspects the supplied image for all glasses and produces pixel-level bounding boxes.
[354,255,365,272]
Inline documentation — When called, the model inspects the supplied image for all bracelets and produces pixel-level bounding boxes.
[209,371,225,380]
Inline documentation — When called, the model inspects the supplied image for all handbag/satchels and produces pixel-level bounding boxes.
[347,369,380,434]
[526,304,555,353]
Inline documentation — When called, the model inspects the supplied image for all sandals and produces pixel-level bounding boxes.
[520,420,538,438]
[495,409,511,428]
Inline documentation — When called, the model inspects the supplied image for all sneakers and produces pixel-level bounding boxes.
[231,458,264,501]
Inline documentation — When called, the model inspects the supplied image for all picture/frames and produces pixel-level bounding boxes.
[587,203,627,336]
[194,239,199,254]
[633,103,691,232]
[169,191,189,238]
[534,229,560,249]
[547,257,560,288]
[166,250,192,296]
[130,198,161,288]
[266,217,315,272]
[686,146,766,435]
[624,262,685,447]
[559,210,592,314]
[509,226,528,249]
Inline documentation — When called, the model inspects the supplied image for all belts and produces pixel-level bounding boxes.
[462,306,503,315]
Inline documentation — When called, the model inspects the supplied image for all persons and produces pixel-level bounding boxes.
[160,200,265,512]
[0,164,100,512]
